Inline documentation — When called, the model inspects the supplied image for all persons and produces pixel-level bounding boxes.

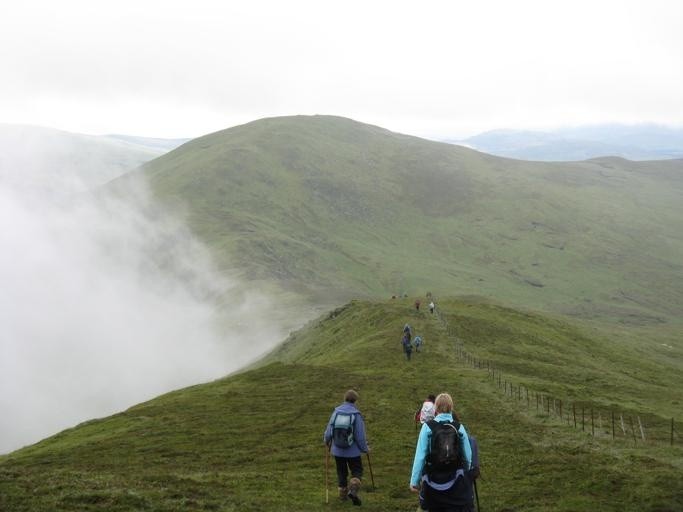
[410,393,474,512]
[451,410,479,481]
[414,394,436,428]
[405,341,413,361]
[415,299,421,311]
[402,334,409,353]
[403,324,412,340]
[429,301,434,314]
[324,389,369,506]
[415,336,421,352]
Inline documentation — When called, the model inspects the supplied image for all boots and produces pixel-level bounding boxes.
[337,478,361,506]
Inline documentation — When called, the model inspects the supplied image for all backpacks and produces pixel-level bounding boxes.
[334,413,356,448]
[425,417,464,468]
[420,402,436,424]
